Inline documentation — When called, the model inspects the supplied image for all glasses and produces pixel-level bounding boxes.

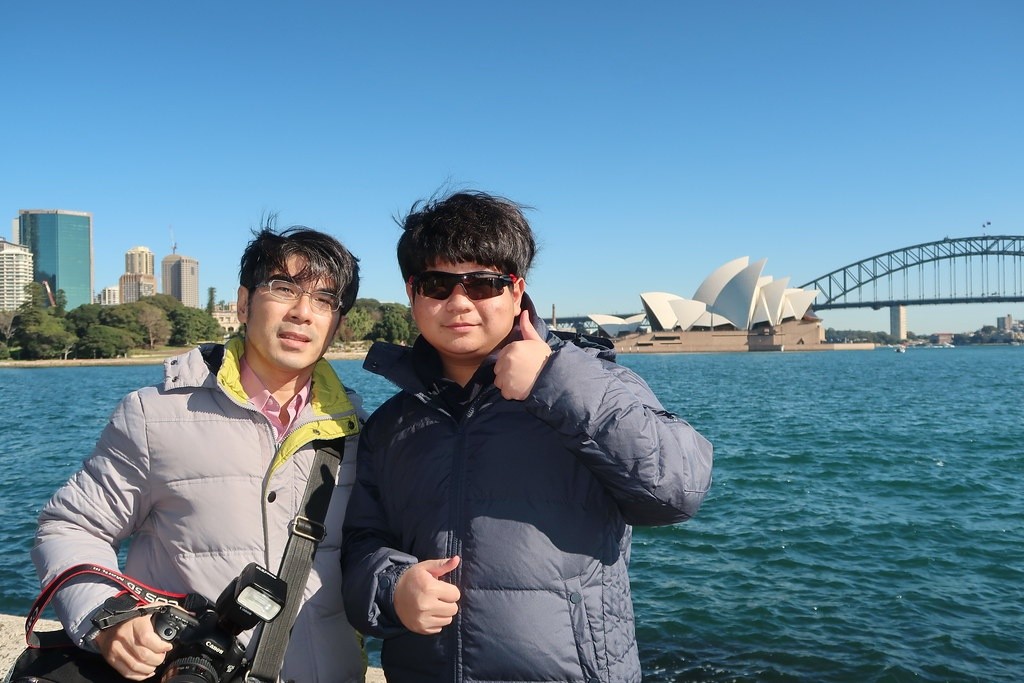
[412,271,515,300]
[254,280,343,311]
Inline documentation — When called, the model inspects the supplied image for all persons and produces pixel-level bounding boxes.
[341,190,714,683]
[31,225,369,683]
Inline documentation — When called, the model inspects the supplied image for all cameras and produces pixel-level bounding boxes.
[150,560,290,683]
